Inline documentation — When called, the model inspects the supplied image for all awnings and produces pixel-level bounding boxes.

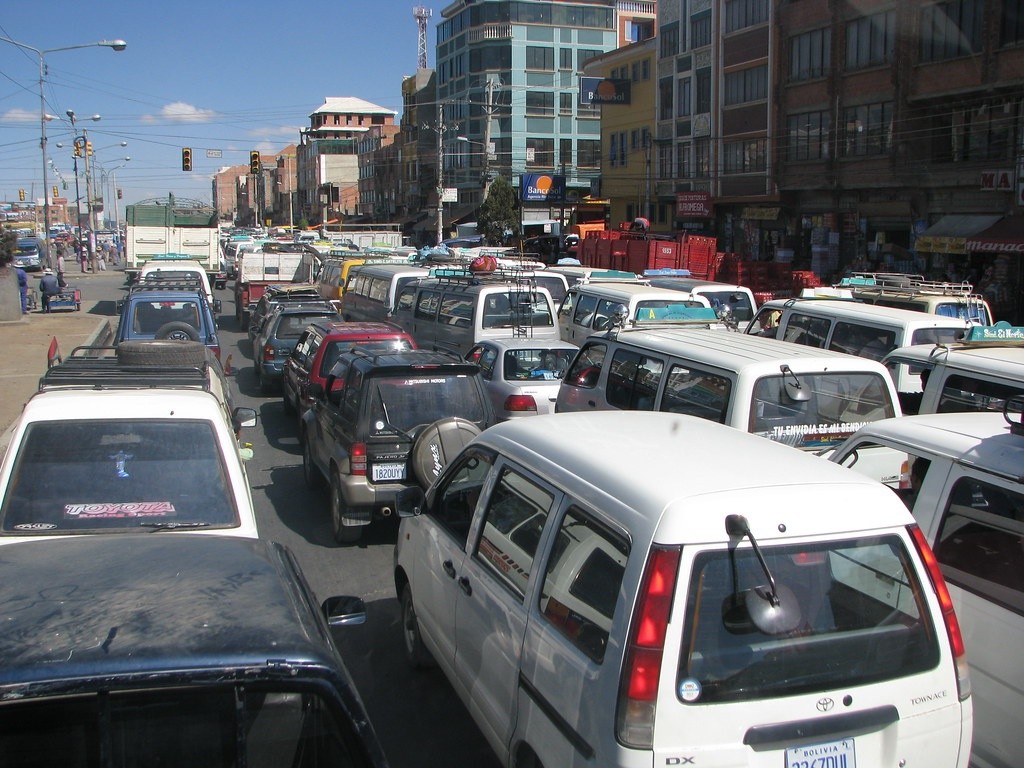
[387,212,428,224]
[967,214,1024,255]
[913,214,1004,255]
[412,203,481,232]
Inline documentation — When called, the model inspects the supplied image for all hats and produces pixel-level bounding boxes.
[14,261,26,267]
[96,246,102,250]
[43,268,53,274]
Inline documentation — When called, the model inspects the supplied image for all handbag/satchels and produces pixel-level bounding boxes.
[469,254,497,274]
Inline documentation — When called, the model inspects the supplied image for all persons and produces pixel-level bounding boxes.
[56,250,68,290]
[81,243,88,273]
[74,237,81,264]
[896,457,931,513]
[97,240,121,266]
[96,246,107,271]
[39,268,68,313]
[13,260,29,315]
[897,368,932,414]
[122,238,127,258]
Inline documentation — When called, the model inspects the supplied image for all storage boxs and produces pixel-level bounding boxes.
[715,232,914,304]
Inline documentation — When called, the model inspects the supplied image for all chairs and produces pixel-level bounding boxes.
[508,355,523,375]
[941,396,979,413]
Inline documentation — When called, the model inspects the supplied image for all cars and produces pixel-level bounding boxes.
[461,337,595,424]
[0,532,395,768]
[0,227,374,411]
[12,224,124,272]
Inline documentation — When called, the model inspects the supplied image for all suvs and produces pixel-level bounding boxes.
[1,338,259,547]
[246,286,415,427]
[297,340,500,547]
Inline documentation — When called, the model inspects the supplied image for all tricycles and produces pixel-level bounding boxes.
[46,282,82,314]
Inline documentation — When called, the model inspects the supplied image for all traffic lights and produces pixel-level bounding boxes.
[182,148,192,171]
[250,151,260,175]
[117,188,122,199]
[87,141,94,157]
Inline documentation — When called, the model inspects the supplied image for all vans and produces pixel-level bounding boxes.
[556,322,916,514]
[814,410,1024,768]
[837,339,1024,443]
[390,409,973,768]
[323,237,1024,497]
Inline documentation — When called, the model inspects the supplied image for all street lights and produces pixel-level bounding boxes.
[456,135,490,203]
[42,110,130,274]
[38,39,126,271]
